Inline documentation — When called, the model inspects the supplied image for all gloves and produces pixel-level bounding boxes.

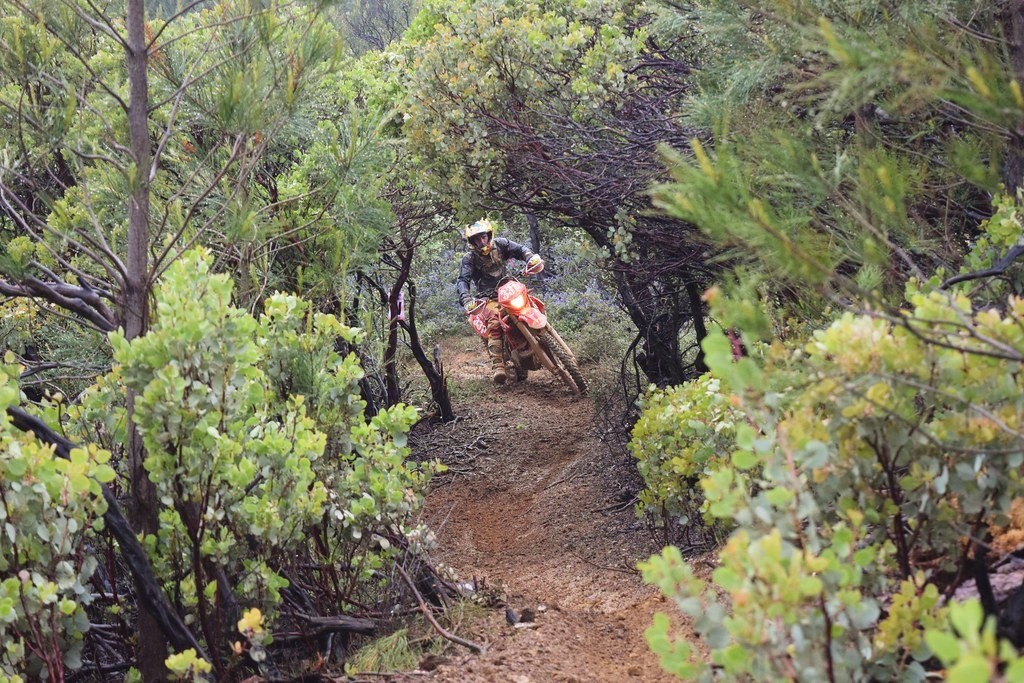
[527,253,544,274]
[462,296,483,315]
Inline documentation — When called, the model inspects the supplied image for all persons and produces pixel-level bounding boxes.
[457,218,544,384]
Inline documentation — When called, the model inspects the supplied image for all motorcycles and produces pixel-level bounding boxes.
[467,257,589,397]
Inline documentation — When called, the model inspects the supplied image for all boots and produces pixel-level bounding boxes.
[488,340,507,383]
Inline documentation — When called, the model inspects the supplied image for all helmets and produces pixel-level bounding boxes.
[465,220,494,255]
